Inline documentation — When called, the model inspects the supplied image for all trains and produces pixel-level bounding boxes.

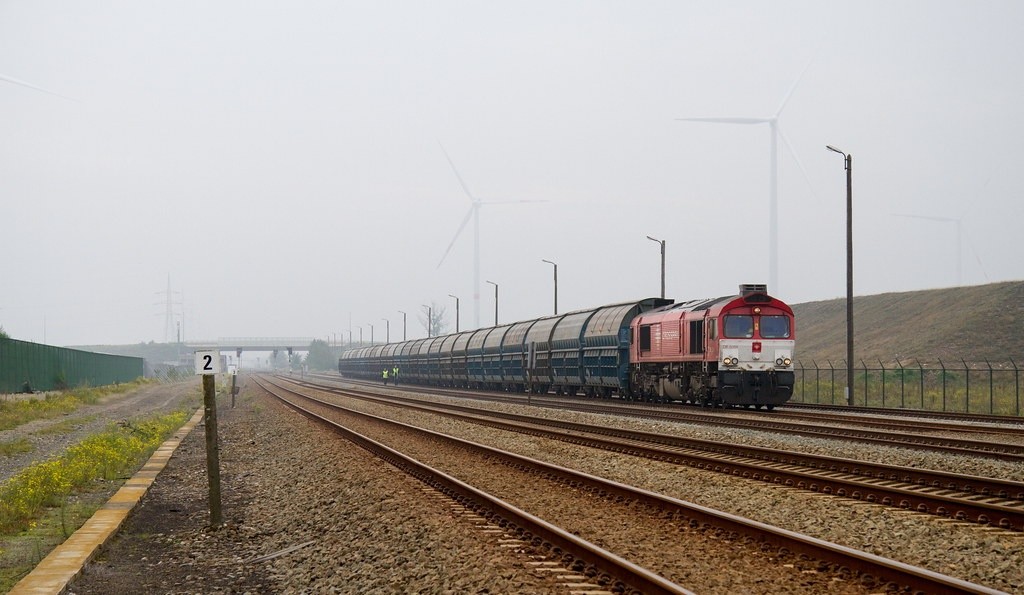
[336,284,796,412]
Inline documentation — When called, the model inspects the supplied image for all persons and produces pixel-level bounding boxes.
[382,367,389,386]
[391,365,400,387]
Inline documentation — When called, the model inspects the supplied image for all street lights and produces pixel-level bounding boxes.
[422,304,431,338]
[449,294,459,333]
[647,236,666,299]
[382,318,389,344]
[398,310,406,341]
[823,143,856,406]
[542,259,558,315]
[487,280,499,326]
[367,324,374,347]
